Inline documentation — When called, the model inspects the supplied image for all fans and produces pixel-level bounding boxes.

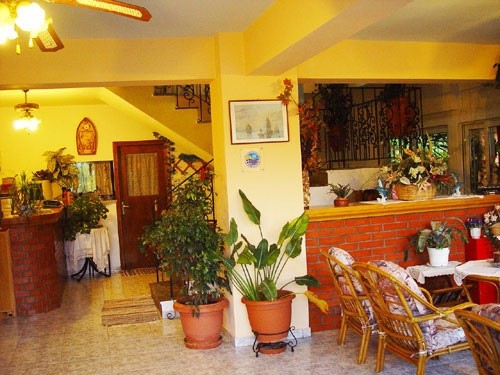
[32,0,152,54]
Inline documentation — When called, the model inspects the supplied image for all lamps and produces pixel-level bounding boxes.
[13,89,41,132]
[0,0,50,45]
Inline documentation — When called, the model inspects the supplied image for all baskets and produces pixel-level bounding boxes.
[392,182,436,201]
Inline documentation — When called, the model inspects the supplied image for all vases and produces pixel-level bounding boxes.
[470,226,482,240]
[493,252,500,263]
[394,183,438,203]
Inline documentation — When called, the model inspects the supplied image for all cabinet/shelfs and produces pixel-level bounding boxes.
[307,185,334,207]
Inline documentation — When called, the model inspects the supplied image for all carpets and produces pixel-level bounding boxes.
[100,295,162,328]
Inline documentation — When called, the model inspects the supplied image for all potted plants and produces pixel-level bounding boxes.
[326,182,355,208]
[57,189,109,242]
[136,167,233,350]
[402,215,469,268]
[207,189,329,357]
[32,169,56,201]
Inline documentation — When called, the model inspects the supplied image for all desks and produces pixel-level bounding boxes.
[453,258,500,305]
[405,259,472,302]
[63,225,113,282]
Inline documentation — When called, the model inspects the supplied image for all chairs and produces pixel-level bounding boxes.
[454,302,500,375]
[352,259,479,375]
[319,246,379,366]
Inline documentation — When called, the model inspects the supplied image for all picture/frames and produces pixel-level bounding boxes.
[228,99,290,145]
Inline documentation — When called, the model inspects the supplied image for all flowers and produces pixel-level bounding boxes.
[378,148,448,191]
[482,203,500,248]
[464,217,483,228]
